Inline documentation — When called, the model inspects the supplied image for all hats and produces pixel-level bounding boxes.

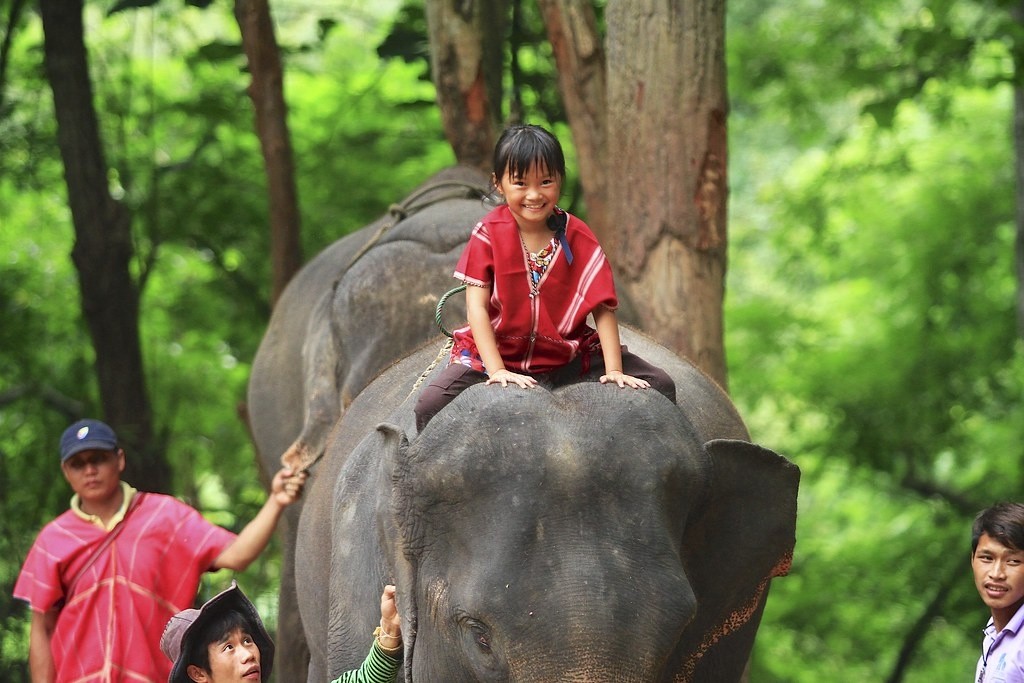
[60,418,117,463]
[159,579,275,683]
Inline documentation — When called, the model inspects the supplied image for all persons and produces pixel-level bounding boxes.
[411,121,677,434]
[13,418,307,683]
[159,583,405,683]
[969,499,1024,683]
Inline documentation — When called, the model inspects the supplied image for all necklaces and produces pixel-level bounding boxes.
[977,623,998,683]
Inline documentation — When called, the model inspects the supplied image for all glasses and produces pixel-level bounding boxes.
[65,453,118,469]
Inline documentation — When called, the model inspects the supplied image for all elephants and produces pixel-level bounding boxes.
[244,162,802,683]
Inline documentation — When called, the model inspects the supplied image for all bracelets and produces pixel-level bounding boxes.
[372,618,402,652]
